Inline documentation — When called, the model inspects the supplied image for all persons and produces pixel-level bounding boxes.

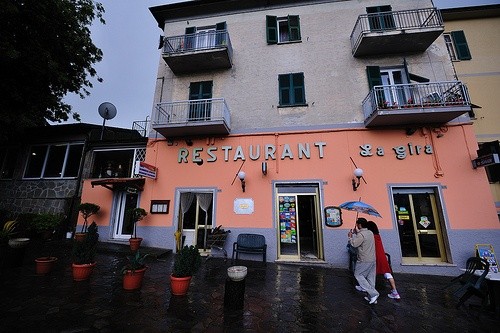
[348,218,380,305]
[355,221,401,299]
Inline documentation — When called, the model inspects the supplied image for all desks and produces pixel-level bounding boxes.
[459,269,500,281]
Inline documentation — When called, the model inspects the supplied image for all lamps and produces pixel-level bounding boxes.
[238,171,245,192]
[262,162,267,175]
[352,168,363,191]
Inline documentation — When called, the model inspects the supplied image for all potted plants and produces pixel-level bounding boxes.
[127,208,148,250]
[171,245,202,296]
[75,203,100,242]
[30,213,61,276]
[72,221,99,281]
[116,250,151,290]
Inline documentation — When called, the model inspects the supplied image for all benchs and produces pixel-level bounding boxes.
[232,233,267,266]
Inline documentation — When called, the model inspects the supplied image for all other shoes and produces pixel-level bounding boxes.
[355,285,366,292]
[363,296,377,304]
[387,292,400,299]
[368,293,379,304]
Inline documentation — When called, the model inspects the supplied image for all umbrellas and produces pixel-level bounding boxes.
[338,197,382,225]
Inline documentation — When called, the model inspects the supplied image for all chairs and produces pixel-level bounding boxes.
[449,257,490,309]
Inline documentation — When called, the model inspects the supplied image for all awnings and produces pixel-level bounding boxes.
[90,178,144,191]
